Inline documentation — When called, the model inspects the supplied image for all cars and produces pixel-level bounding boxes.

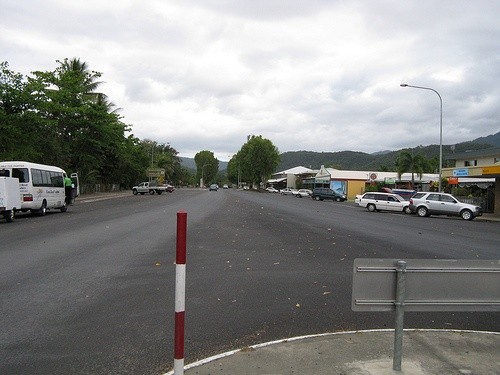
[223,184,228,189]
[209,184,219,191]
[292,188,313,198]
[355,193,362,204]
[280,188,298,195]
[163,184,175,192]
[266,187,279,193]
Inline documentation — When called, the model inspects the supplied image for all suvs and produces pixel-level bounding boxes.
[408,191,483,221]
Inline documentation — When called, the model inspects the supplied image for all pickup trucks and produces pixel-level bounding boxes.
[132,181,167,195]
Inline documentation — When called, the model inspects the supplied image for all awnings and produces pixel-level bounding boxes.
[268,177,287,184]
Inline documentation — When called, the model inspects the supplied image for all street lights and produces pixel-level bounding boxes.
[399,84,443,192]
[151,142,170,181]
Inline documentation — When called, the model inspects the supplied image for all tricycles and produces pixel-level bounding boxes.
[0,177,22,222]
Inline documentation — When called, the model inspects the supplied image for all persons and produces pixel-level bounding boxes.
[64,174,72,206]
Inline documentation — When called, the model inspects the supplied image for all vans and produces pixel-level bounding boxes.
[0,160,80,215]
[313,188,347,202]
[358,191,412,214]
